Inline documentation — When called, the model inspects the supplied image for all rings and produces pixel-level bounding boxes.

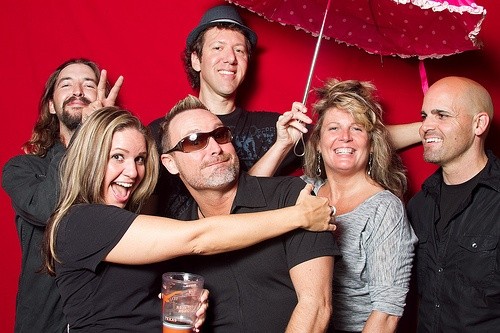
[329,204,336,217]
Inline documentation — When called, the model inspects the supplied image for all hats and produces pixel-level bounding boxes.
[185,6,258,51]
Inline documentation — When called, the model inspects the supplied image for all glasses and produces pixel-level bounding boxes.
[167,126,233,153]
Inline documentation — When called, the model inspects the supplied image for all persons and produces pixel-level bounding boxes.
[248,72,417,333]
[38,107,337,333]
[2,57,124,333]
[400,75,500,333]
[82,68,343,333]
[145,3,423,333]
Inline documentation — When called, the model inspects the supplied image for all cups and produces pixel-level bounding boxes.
[162,272,204,333]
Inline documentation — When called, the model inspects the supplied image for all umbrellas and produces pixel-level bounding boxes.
[224,0,487,122]
